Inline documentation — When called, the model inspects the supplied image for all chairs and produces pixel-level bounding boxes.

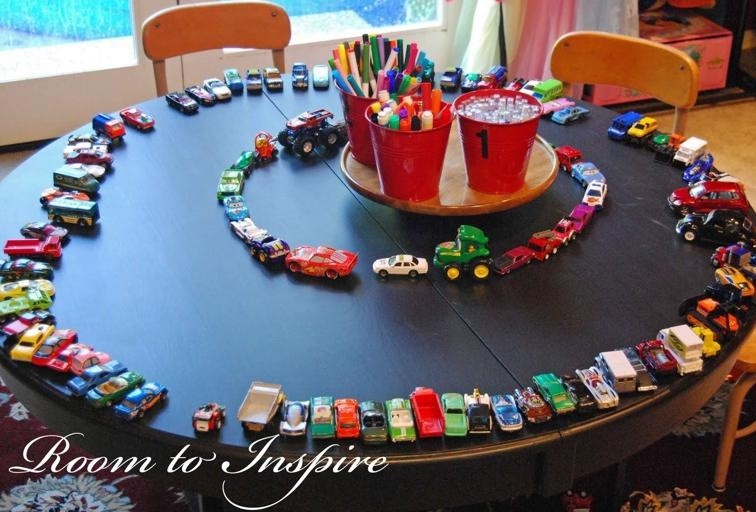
[712,327,756,492]
[141,1,290,97]
[548,32,698,136]
[0,372,190,512]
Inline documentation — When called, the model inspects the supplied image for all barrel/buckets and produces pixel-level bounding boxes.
[334,79,419,165]
[365,98,458,200]
[453,87,543,194]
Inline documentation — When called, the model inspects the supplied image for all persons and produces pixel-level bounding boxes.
[0,62,756,446]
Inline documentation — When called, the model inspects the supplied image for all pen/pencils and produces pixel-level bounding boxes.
[327,34,450,132]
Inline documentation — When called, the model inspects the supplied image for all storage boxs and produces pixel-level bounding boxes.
[587,5,728,107]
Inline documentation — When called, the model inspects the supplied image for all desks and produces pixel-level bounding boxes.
[0,69,756,512]
